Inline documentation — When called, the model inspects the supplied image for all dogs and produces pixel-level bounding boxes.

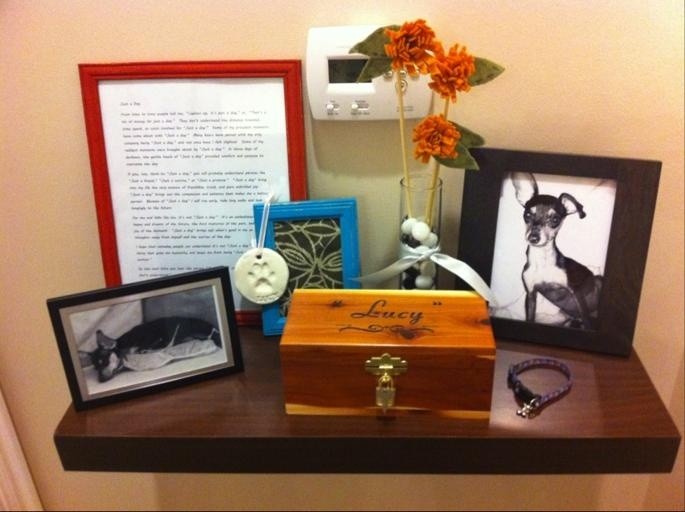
[510,170,603,330]
[78,316,222,382]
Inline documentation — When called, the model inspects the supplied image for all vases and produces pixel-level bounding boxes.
[395,172,443,289]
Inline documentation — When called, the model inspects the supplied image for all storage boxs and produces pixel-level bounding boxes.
[279,288,498,419]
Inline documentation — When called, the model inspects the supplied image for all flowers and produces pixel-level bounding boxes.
[344,18,507,237]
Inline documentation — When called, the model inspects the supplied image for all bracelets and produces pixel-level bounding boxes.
[508,358,574,416]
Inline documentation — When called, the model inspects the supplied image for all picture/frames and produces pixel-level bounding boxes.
[78,58,309,327]
[250,199,360,339]
[45,265,245,414]
[458,146,661,360]
